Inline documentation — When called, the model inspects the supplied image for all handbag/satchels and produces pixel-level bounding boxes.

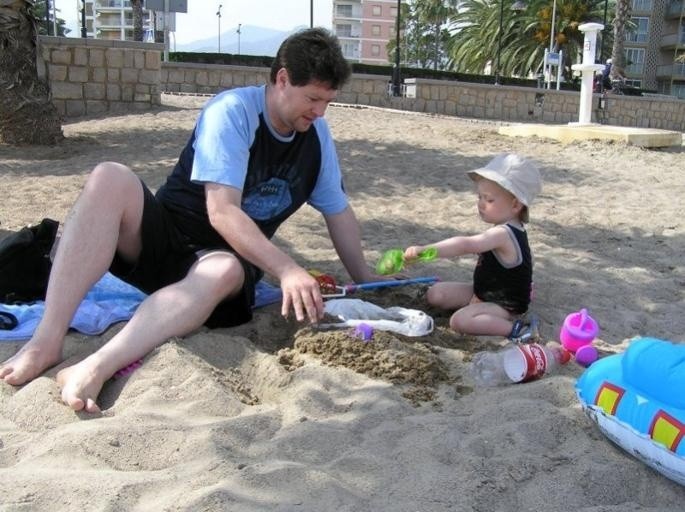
[0,218,59,307]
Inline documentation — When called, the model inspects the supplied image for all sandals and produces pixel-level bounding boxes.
[508,314,538,346]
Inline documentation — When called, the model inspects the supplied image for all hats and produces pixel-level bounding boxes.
[465,151,543,224]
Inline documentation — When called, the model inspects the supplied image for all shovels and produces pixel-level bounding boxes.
[375,247,437,277]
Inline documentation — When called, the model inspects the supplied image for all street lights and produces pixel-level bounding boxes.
[237,23,243,55]
[217,4,224,54]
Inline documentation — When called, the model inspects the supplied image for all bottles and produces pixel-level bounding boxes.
[471,344,571,388]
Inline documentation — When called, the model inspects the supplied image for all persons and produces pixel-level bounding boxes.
[403,152,541,345]
[0,27,411,415]
[598,58,628,94]
